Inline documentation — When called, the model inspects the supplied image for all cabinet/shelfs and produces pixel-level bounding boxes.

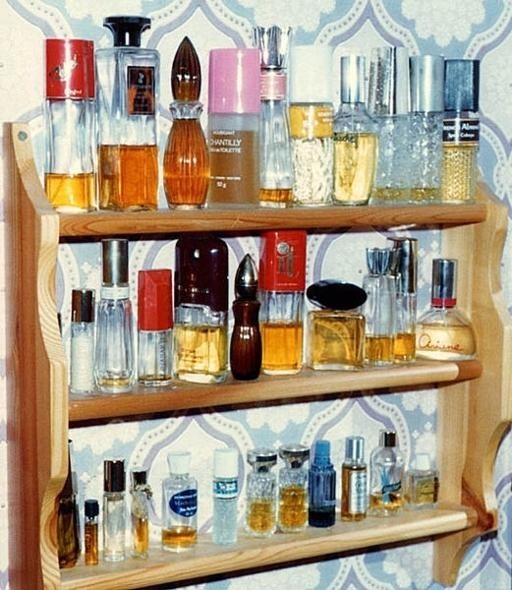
[4,121,511,590]
